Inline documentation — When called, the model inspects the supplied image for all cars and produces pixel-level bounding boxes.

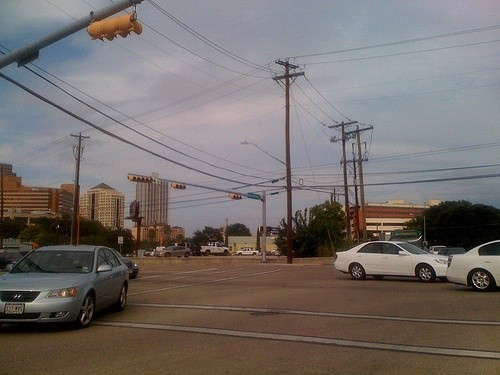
[0,244,139,329]
[333,239,500,293]
[144,241,193,258]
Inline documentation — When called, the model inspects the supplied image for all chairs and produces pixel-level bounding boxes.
[79,256,91,266]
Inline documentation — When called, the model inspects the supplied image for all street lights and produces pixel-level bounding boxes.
[409,212,426,242]
[240,141,292,264]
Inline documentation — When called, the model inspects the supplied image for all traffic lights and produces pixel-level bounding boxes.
[127,173,154,183]
[170,182,187,189]
[228,192,242,200]
[86,12,143,43]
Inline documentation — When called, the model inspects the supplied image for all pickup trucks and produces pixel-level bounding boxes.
[199,242,230,255]
[235,247,260,256]
